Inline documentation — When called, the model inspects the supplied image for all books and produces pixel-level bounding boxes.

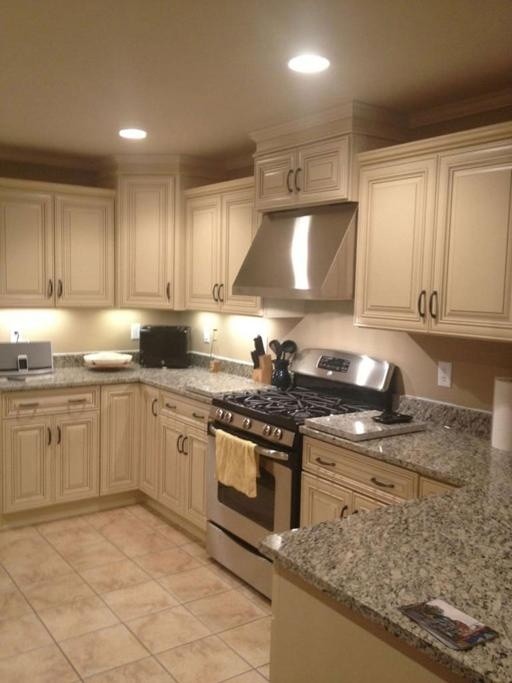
[400,599,499,651]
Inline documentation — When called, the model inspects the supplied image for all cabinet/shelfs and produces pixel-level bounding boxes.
[140,384,161,497]
[100,384,140,495]
[1,385,101,515]
[355,143,511,340]
[182,186,263,317]
[117,173,175,311]
[0,186,116,308]
[419,477,457,499]
[157,394,210,527]
[253,134,353,212]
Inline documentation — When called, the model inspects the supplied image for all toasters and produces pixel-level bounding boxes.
[139,323,193,368]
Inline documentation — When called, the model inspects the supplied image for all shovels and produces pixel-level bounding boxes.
[269,340,296,361]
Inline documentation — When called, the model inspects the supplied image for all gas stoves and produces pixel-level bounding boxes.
[211,386,360,446]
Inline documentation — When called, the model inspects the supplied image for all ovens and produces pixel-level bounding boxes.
[203,419,297,601]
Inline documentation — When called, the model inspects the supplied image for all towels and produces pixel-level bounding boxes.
[214,429,262,499]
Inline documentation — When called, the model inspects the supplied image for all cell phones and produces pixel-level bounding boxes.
[17,355,29,375]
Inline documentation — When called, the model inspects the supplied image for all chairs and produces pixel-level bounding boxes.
[299,434,419,527]
